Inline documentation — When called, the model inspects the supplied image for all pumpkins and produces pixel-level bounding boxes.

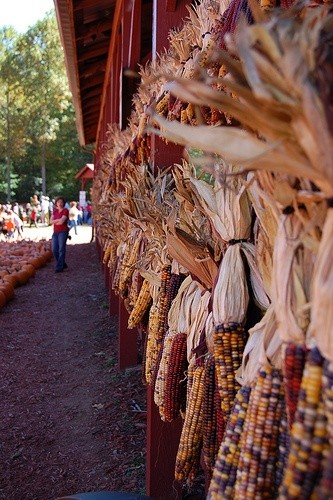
[0,237,53,309]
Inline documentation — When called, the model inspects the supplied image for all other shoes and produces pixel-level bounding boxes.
[64,263,68,269]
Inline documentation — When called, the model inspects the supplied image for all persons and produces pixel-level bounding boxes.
[0,194,93,240]
[49,195,70,274]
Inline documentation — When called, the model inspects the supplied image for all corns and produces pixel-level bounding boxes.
[94,0,333,500]
